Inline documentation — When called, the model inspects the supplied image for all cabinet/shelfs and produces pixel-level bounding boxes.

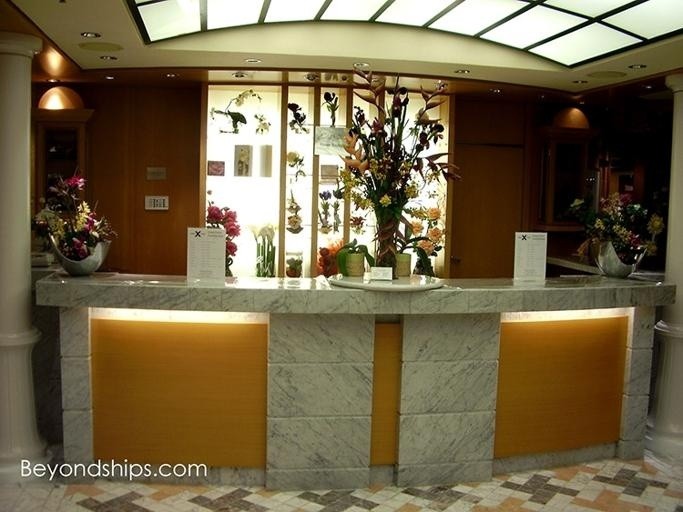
[200,83,453,278]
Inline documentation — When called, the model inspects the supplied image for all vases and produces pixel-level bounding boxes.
[47,230,114,276]
[412,257,435,276]
[589,238,649,277]
[374,204,402,266]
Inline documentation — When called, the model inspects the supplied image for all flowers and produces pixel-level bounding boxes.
[249,221,278,277]
[285,189,302,230]
[322,90,340,127]
[288,101,311,136]
[566,155,666,268]
[318,191,333,224]
[331,188,343,215]
[33,166,120,262]
[287,152,308,180]
[207,199,240,272]
[340,66,463,226]
[410,204,443,276]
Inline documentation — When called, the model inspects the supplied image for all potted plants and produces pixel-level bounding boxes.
[338,240,375,282]
[395,224,436,278]
